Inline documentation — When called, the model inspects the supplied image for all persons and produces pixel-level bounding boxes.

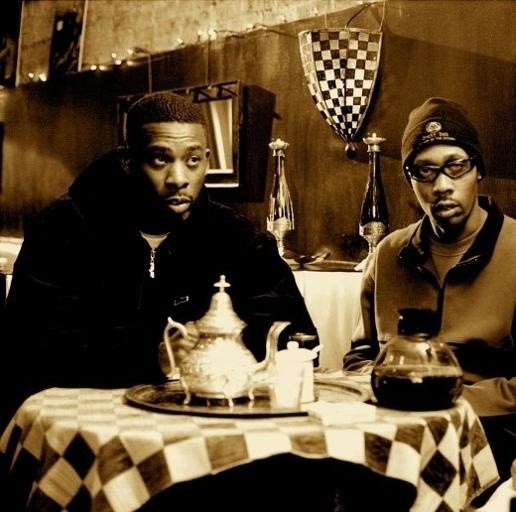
[1,90,320,432]
[342,96,515,472]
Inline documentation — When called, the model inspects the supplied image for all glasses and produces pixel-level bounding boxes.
[405,154,477,183]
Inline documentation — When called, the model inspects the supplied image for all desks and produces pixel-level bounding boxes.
[290,268,366,370]
[0,370,500,512]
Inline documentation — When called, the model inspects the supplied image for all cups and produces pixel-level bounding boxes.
[273,341,315,407]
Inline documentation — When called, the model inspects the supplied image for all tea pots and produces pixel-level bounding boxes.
[163,274,290,407]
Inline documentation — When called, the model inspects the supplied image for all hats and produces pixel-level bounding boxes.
[400,96,487,184]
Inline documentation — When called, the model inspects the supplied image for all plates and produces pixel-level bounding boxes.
[303,260,361,272]
[123,379,372,417]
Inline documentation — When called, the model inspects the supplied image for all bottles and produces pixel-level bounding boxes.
[358,129,390,256]
[265,138,299,270]
[372,306,464,410]
